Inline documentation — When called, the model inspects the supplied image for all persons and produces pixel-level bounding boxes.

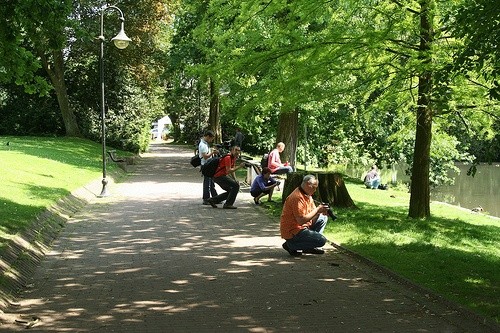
[364,165,381,189]
[207,144,246,209]
[268,142,293,174]
[198,130,222,206]
[279,174,330,256]
[250,167,281,205]
[234,127,245,149]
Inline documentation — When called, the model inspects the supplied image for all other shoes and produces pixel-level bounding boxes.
[202,199,222,205]
[223,203,237,208]
[303,247,325,254]
[282,242,302,256]
[268,198,274,201]
[254,198,260,204]
[208,197,218,207]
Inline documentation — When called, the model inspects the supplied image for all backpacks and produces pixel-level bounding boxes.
[199,154,232,176]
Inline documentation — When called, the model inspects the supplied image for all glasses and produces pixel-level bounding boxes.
[234,148,241,152]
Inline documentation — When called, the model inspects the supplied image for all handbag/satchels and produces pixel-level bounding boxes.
[190,154,201,168]
[260,157,268,168]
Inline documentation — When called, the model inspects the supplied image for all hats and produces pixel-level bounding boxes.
[229,140,240,147]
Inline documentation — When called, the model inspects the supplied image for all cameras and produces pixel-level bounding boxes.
[278,182,280,185]
[244,163,252,168]
[321,203,337,221]
